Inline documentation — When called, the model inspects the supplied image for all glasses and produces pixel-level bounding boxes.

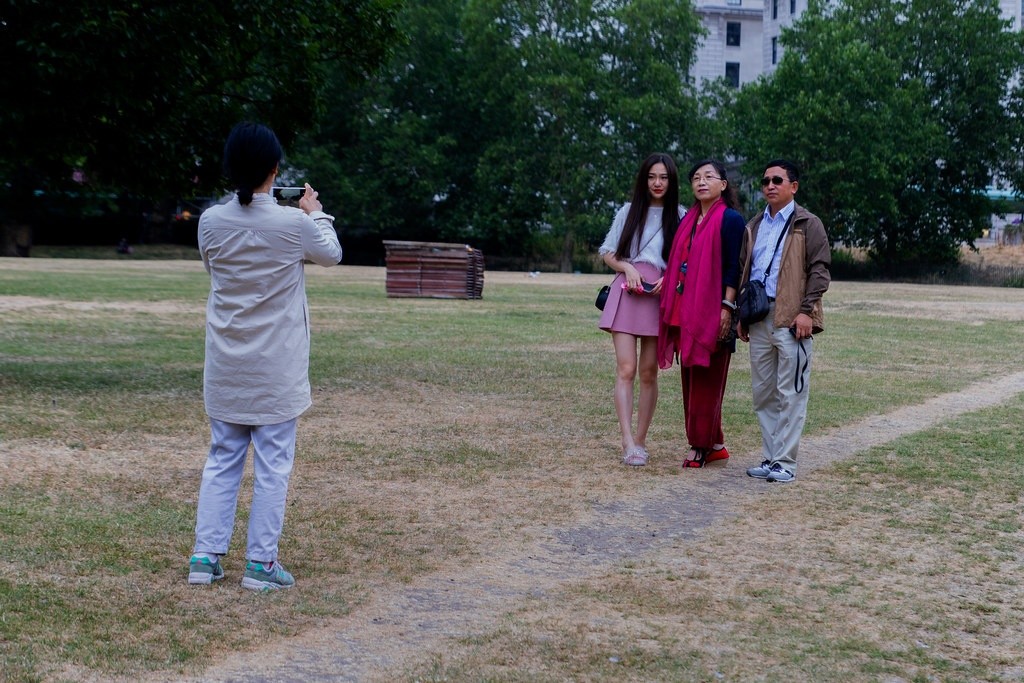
[676,262,687,294]
[761,177,794,185]
[690,175,723,182]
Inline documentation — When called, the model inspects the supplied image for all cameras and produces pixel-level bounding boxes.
[789,327,796,338]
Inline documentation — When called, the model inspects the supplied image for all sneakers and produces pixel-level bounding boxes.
[188,554,225,584]
[746,461,771,478]
[766,464,796,482]
[241,561,295,589]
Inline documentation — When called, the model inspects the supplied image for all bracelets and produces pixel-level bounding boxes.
[723,300,737,315]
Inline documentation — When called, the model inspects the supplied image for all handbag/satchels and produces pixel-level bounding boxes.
[595,286,610,311]
[736,279,769,324]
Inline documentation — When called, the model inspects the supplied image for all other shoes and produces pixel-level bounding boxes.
[683,447,707,467]
[705,447,729,464]
[622,450,645,466]
[636,447,649,461]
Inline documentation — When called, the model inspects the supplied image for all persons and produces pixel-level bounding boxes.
[657,159,747,468]
[187,123,343,591]
[597,151,689,466]
[735,159,831,483]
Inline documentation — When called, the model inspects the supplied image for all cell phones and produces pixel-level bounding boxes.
[271,187,305,208]
[640,280,655,293]
